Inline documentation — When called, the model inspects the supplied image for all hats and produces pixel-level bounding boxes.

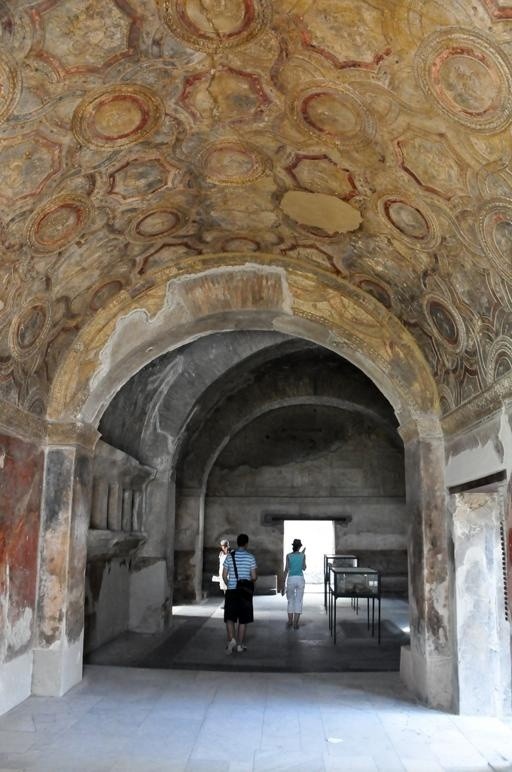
[292,539,302,546]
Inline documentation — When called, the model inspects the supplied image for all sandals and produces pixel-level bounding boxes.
[288,621,299,629]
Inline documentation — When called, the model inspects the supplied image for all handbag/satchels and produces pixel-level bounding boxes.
[237,579,254,596]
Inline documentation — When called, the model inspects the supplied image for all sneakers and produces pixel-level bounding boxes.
[225,638,247,655]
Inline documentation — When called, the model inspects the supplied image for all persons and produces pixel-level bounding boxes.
[219,539,229,609]
[282,539,306,629]
[222,534,257,655]
